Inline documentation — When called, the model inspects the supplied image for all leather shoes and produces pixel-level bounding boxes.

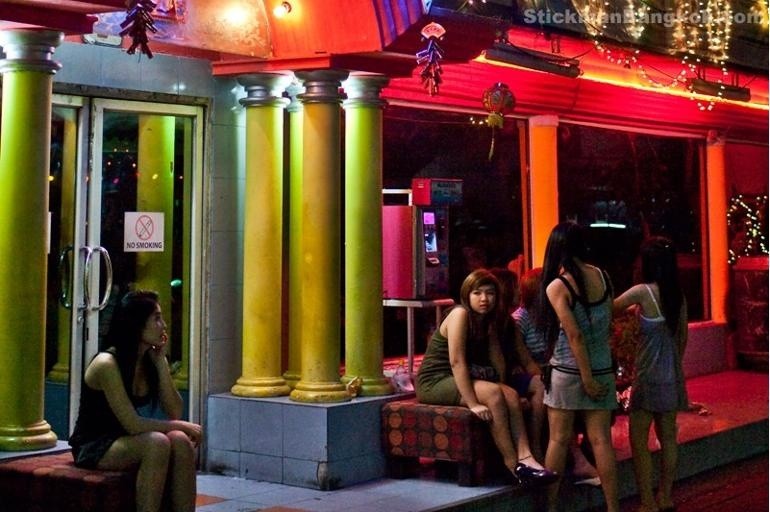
[568,471,602,491]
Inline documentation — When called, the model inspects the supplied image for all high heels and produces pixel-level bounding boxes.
[506,459,563,494]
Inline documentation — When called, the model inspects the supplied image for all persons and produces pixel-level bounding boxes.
[615,237,688,512]
[69,290,204,512]
[510,268,602,486]
[536,220,619,511]
[488,268,549,466]
[416,269,560,493]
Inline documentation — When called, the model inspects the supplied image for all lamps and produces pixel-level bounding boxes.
[482,31,583,79]
[272,1,292,19]
[685,65,751,102]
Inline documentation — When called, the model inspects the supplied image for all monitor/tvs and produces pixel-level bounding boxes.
[424,231,438,254]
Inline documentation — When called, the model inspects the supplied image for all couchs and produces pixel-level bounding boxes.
[383,397,532,488]
[0,450,138,512]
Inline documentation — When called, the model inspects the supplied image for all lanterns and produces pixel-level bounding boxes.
[483,81,516,160]
